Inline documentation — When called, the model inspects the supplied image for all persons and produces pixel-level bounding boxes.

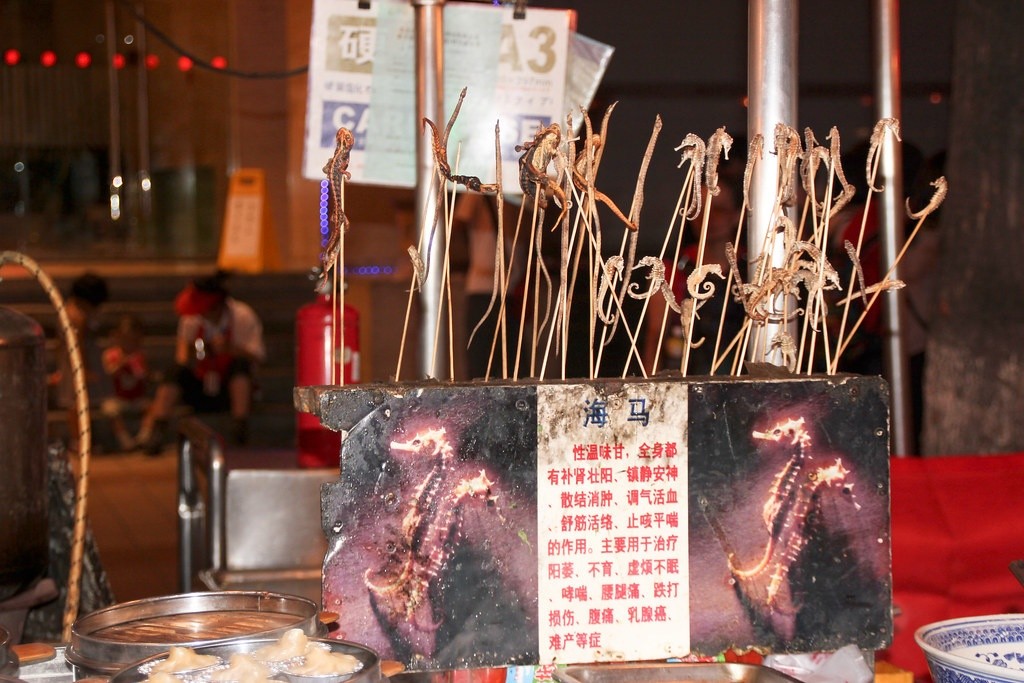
[139,267,265,452]
[56,271,135,453]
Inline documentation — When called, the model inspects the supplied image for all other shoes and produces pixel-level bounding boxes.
[24,415,265,463]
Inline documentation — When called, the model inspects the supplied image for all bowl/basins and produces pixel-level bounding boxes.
[915,612,1024,682]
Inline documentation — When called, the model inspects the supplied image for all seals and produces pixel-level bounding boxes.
[646,268,708,315]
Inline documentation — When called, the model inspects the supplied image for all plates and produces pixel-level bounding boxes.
[106,635,385,683]
[549,658,804,682]
[64,589,325,673]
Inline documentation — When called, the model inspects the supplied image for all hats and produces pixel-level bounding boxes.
[170,282,229,317]
